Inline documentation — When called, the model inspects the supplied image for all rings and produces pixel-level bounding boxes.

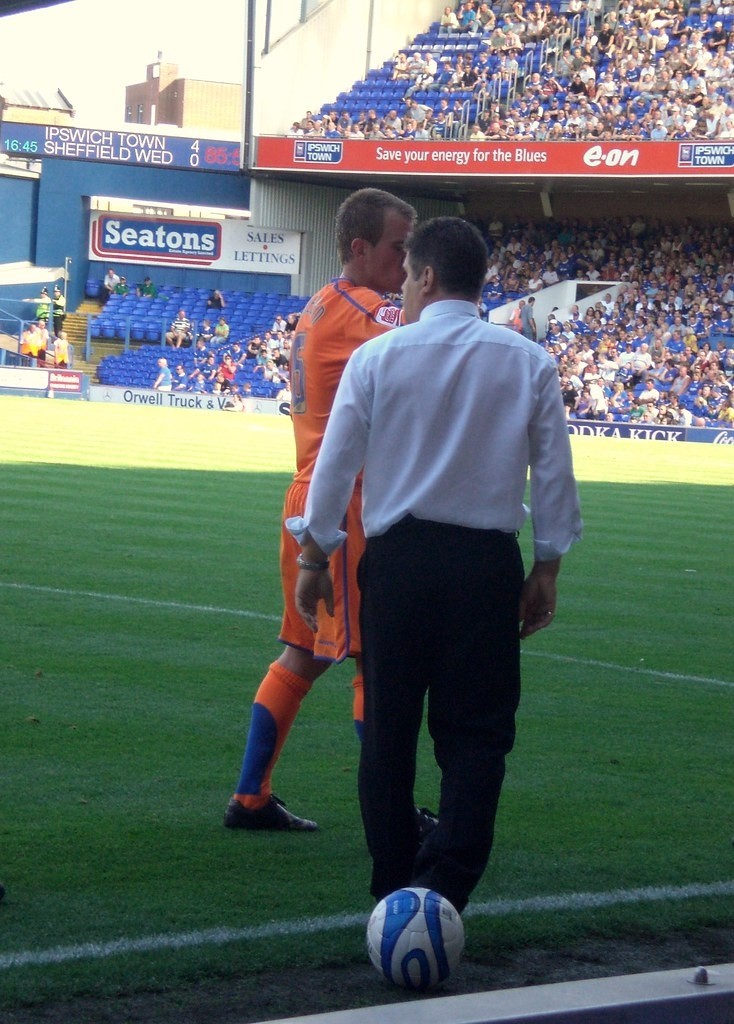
[548,612,552,614]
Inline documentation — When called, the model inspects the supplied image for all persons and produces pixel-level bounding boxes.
[285,217,583,915]
[223,187,438,834]
[153,289,302,401]
[288,1,734,429]
[101,269,157,297]
[19,283,71,368]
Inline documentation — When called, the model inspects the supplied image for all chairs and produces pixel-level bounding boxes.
[85,0,734,429]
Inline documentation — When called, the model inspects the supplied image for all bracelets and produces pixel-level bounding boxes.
[297,554,329,570]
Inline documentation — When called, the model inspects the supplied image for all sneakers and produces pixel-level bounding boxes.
[223,793,318,833]
[415,806,438,845]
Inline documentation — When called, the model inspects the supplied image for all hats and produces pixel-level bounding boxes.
[119,276,126,281]
[548,319,557,324]
[565,381,573,386]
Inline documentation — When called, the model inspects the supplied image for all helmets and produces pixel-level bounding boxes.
[52,285,61,292]
[40,286,48,294]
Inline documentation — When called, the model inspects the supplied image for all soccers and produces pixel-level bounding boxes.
[363,885,466,992]
